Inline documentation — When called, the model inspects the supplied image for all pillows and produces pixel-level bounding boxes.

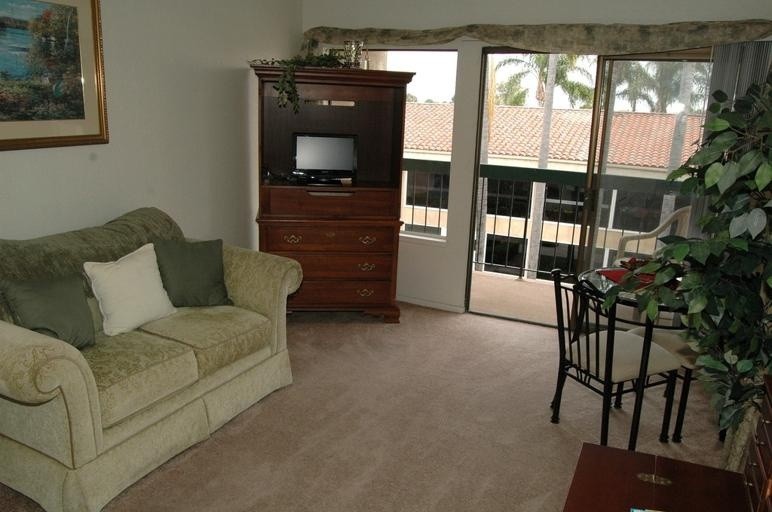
[149,235,237,308]
[0,270,96,353]
[81,241,179,338]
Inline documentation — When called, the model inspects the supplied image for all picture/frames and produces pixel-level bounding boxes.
[0,0,110,152]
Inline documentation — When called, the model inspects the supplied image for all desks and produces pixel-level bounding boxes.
[548,265,734,449]
[557,440,751,512]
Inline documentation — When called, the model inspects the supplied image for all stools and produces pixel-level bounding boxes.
[613,325,733,447]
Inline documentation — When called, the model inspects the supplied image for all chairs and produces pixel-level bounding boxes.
[615,205,692,267]
[545,266,681,448]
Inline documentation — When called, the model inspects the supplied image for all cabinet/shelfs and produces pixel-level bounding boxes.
[244,59,417,324]
[741,374,771,510]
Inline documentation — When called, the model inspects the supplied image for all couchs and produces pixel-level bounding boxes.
[1,205,306,511]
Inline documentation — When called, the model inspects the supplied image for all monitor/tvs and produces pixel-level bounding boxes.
[290,132,358,186]
